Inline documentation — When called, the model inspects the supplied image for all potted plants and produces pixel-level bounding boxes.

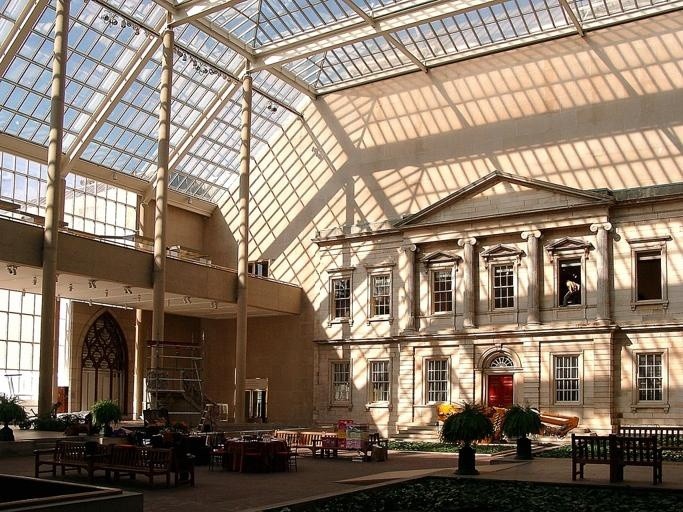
[500,401,542,460]
[91,399,121,435]
[438,403,494,474]
[0,392,28,441]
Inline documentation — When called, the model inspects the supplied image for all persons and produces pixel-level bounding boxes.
[560,279,580,307]
[51,402,61,418]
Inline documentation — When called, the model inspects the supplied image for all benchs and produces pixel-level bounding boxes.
[274,430,338,459]
[33,439,174,487]
[571,432,663,485]
[618,423,683,463]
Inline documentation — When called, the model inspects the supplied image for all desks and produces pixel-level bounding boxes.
[225,438,289,474]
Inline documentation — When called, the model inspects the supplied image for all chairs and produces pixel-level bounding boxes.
[275,441,299,477]
[367,434,389,449]
[209,437,226,468]
